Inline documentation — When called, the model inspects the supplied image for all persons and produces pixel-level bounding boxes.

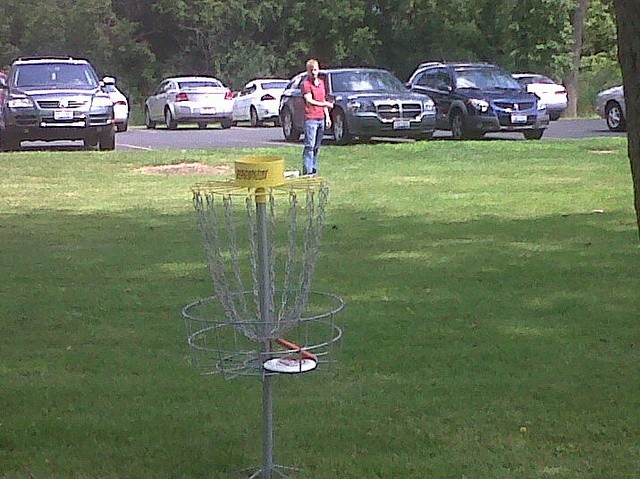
[301,59,334,177]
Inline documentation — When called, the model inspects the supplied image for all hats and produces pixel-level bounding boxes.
[101,85,128,132]
[513,74,567,121]
[232,80,291,127]
[145,77,233,130]
[278,68,436,147]
[593,85,627,131]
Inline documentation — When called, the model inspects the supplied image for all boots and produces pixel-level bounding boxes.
[411,63,549,140]
[0,55,115,152]
